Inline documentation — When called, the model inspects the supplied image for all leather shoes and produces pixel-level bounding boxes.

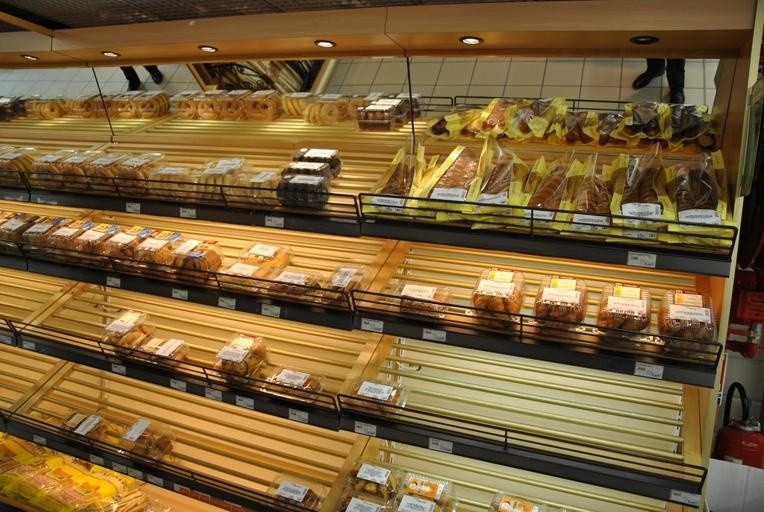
[669,88,684,105]
[151,71,163,84]
[632,72,654,90]
[127,86,138,91]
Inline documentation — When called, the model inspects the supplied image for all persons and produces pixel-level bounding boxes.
[120,66,164,92]
[632,58,686,106]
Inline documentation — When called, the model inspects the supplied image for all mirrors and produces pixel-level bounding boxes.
[1,46,738,113]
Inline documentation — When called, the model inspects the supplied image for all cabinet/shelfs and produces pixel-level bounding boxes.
[2,0,764,512]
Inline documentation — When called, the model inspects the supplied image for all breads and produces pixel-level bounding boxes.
[25,97,68,119]
[67,90,169,119]
[170,89,280,121]
[223,243,358,307]
[277,147,341,209]
[372,148,718,224]
[282,91,420,130]
[400,285,451,320]
[432,98,719,154]
[149,161,280,207]
[333,460,545,512]
[0,432,150,512]
[32,155,153,193]
[472,267,717,357]
[105,311,320,404]
[59,411,174,462]
[265,475,321,512]
[0,211,222,279]
[0,95,38,121]
[0,145,35,181]
